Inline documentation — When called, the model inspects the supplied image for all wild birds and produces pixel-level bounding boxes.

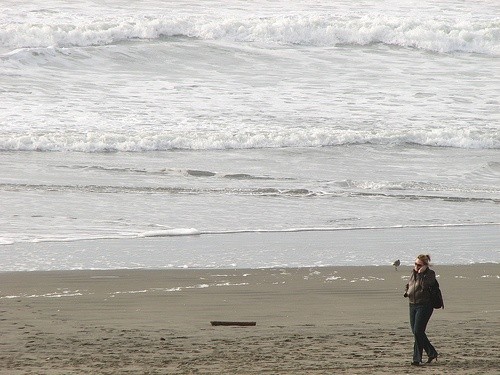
[393,259,400,270]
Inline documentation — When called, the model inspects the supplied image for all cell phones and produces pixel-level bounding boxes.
[419,265,427,272]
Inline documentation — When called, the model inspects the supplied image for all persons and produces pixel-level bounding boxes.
[404,255,438,366]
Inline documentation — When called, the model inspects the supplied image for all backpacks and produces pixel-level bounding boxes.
[423,268,445,310]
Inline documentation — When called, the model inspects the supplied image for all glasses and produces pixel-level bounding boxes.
[415,262,425,268]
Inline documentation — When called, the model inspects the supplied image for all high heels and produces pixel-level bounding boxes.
[410,360,422,366]
[426,351,438,363]
